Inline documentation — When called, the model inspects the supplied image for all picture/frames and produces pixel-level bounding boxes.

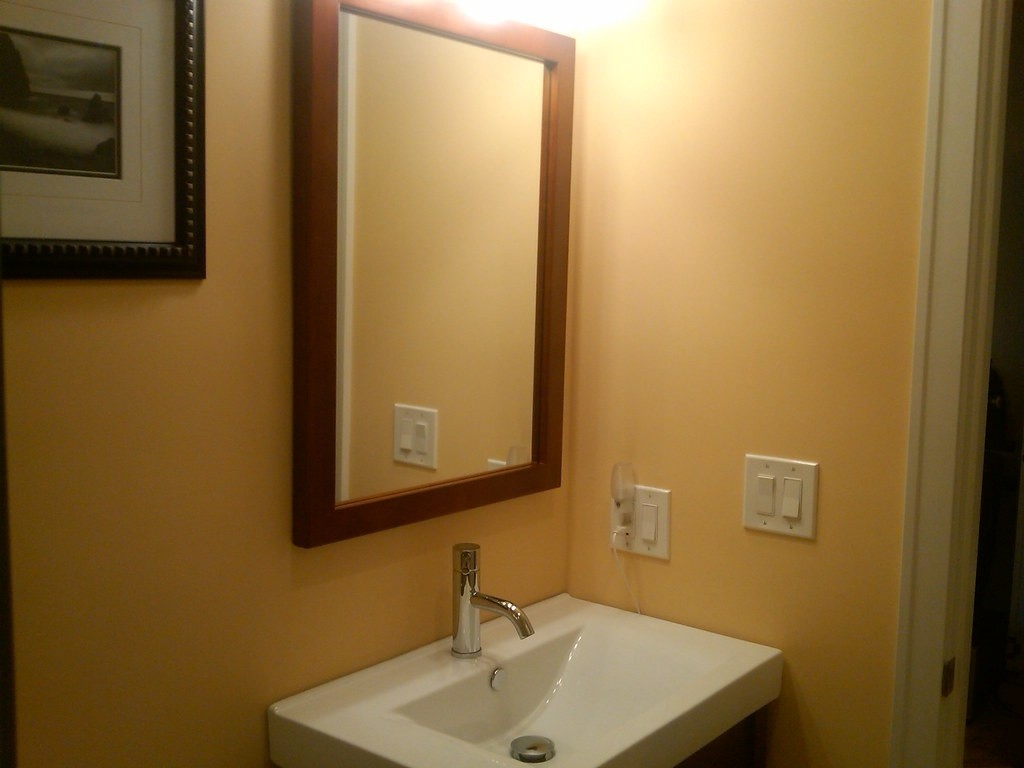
[1,1,206,280]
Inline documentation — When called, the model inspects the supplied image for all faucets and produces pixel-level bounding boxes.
[449,541,536,657]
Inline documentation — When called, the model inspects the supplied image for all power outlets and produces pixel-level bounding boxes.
[609,484,675,562]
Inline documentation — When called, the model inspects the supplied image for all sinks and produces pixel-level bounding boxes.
[264,591,786,768]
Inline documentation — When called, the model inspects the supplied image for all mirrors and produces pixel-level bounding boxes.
[295,0,576,549]
